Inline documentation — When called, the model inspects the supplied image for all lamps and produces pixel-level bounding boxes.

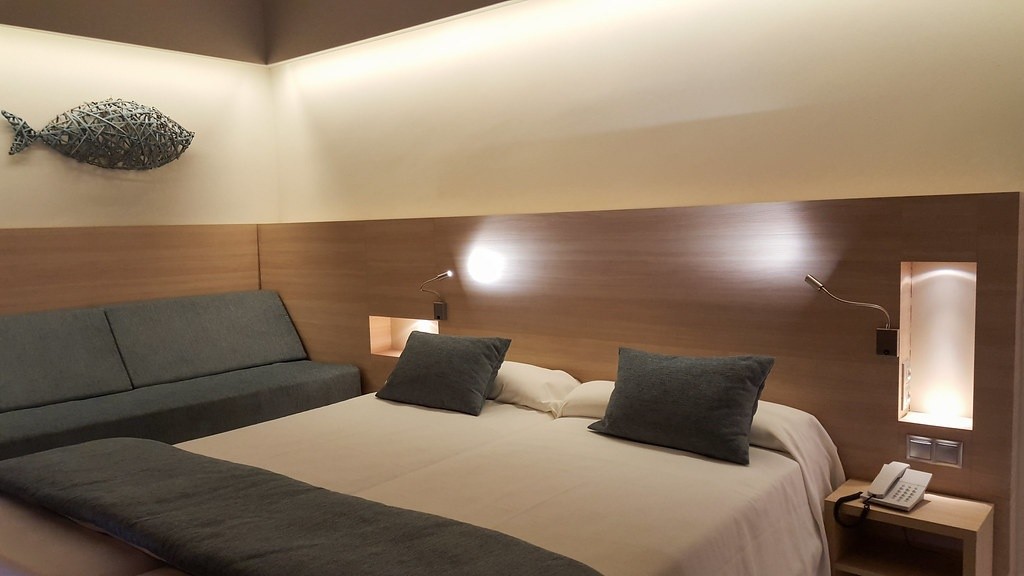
[801,275,901,357]
[417,270,455,320]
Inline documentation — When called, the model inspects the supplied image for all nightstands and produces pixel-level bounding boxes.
[823,479,994,576]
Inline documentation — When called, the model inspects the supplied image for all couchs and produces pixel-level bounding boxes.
[0,291,363,469]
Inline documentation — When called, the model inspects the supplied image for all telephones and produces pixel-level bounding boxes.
[859,459,933,512]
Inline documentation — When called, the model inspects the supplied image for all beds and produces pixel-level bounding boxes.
[0,359,844,576]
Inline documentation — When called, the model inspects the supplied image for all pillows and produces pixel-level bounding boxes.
[489,360,583,416]
[557,380,817,456]
[586,346,775,467]
[375,331,511,417]
